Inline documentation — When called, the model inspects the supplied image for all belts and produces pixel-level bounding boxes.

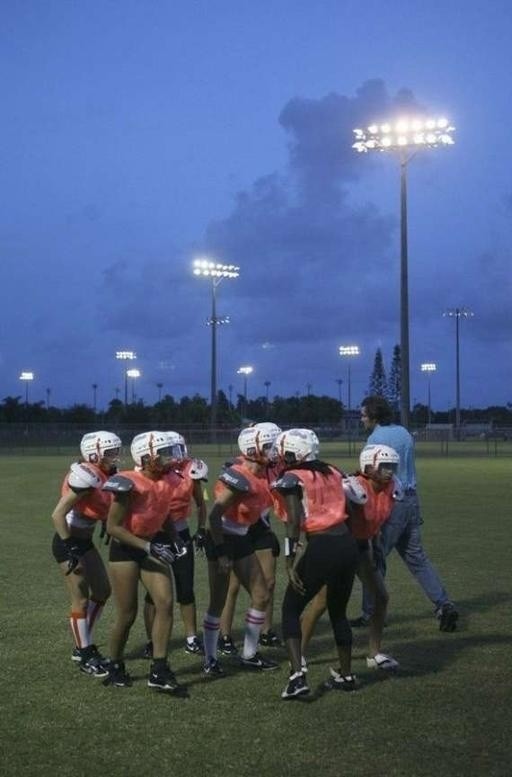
[405,488,416,496]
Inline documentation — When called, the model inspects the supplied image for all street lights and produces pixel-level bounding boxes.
[190,257,241,401]
[263,379,271,397]
[335,378,345,403]
[155,381,166,399]
[348,112,455,429]
[420,362,436,425]
[125,366,141,402]
[337,344,359,412]
[46,385,52,408]
[92,382,98,410]
[439,305,474,426]
[114,348,135,403]
[19,370,36,405]
[236,364,253,401]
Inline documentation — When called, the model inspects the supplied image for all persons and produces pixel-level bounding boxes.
[51,420,401,699]
[349,395,459,637]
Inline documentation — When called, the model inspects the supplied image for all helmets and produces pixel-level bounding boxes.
[166,430,188,458]
[131,431,177,473]
[358,444,400,483]
[238,422,319,466]
[79,431,123,476]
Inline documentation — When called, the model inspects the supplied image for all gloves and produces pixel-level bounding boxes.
[147,541,176,567]
[63,544,80,576]
[99,522,110,545]
[175,539,191,563]
[193,531,205,552]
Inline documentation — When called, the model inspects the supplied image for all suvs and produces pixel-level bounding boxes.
[455,418,509,442]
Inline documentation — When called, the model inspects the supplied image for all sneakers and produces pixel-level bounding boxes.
[185,635,238,677]
[239,652,281,671]
[258,630,286,647]
[350,617,387,627]
[72,644,126,687]
[439,601,460,633]
[366,655,400,670]
[281,656,360,700]
[148,671,187,692]
[143,642,153,658]
